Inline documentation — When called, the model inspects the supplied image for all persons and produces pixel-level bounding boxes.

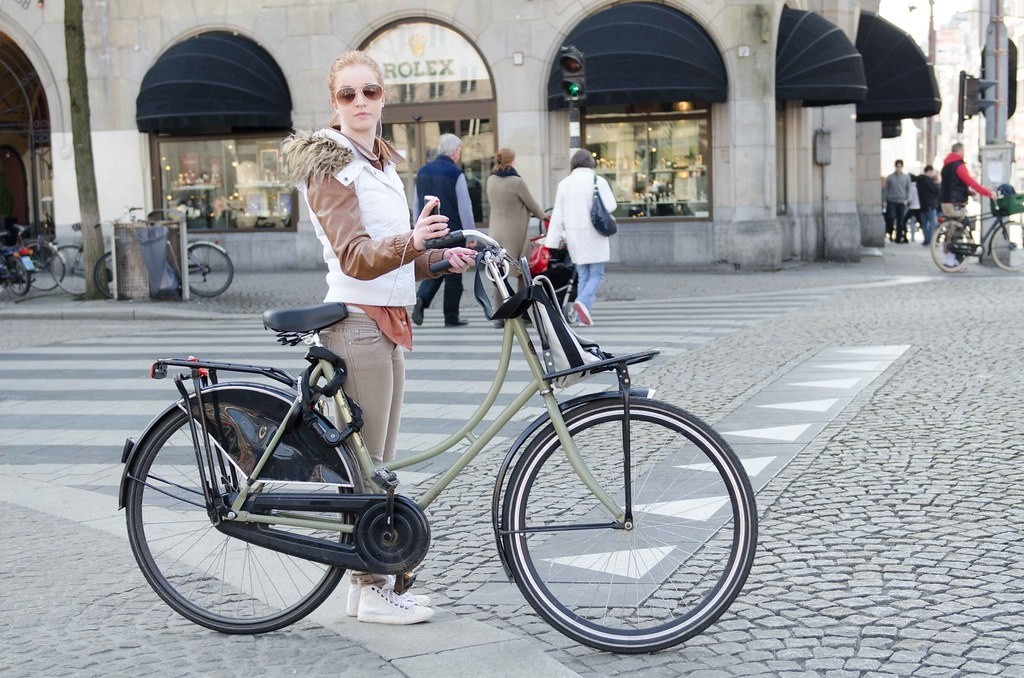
[915,165,939,246]
[411,133,477,325]
[940,143,998,265]
[280,50,478,625]
[486,148,547,329]
[901,174,923,243]
[544,149,617,326]
[883,159,912,242]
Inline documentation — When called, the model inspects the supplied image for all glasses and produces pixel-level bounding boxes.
[331,84,384,107]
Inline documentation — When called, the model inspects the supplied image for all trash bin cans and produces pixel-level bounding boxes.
[110,207,191,301]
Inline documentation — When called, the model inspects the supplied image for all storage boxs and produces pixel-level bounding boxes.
[990,192,1024,215]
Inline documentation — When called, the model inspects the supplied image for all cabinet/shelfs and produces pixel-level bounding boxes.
[233,139,291,229]
[168,140,227,229]
[586,121,647,216]
[646,119,708,217]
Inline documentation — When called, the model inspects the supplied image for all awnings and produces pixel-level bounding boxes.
[547,1,728,112]
[136,30,294,134]
[854,9,942,122]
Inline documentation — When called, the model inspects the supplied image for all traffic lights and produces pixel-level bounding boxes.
[958,71,999,133]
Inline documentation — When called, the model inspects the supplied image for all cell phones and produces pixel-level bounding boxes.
[423,196,440,232]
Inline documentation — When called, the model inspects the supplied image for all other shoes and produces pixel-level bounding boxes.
[445,317,470,327]
[494,319,504,328]
[412,297,426,325]
[943,254,960,266]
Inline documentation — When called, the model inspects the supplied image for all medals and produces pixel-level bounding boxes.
[775,8,868,109]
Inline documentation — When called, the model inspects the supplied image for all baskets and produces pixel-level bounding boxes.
[990,193,1024,217]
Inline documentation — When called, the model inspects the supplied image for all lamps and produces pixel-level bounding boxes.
[754,4,770,43]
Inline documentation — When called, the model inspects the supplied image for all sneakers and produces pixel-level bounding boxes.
[358,581,434,625]
[347,578,430,617]
[573,301,594,327]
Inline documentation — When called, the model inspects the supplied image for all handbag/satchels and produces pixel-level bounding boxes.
[591,174,617,236]
[525,235,550,273]
[472,250,602,387]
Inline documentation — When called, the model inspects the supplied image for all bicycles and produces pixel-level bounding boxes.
[930,191,1024,273]
[1,208,235,301]
[117,230,759,655]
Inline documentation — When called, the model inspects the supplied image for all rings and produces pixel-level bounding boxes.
[427,226,432,233]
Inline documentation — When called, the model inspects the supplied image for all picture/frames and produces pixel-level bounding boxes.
[260,149,278,171]
[277,192,291,215]
[246,192,266,217]
